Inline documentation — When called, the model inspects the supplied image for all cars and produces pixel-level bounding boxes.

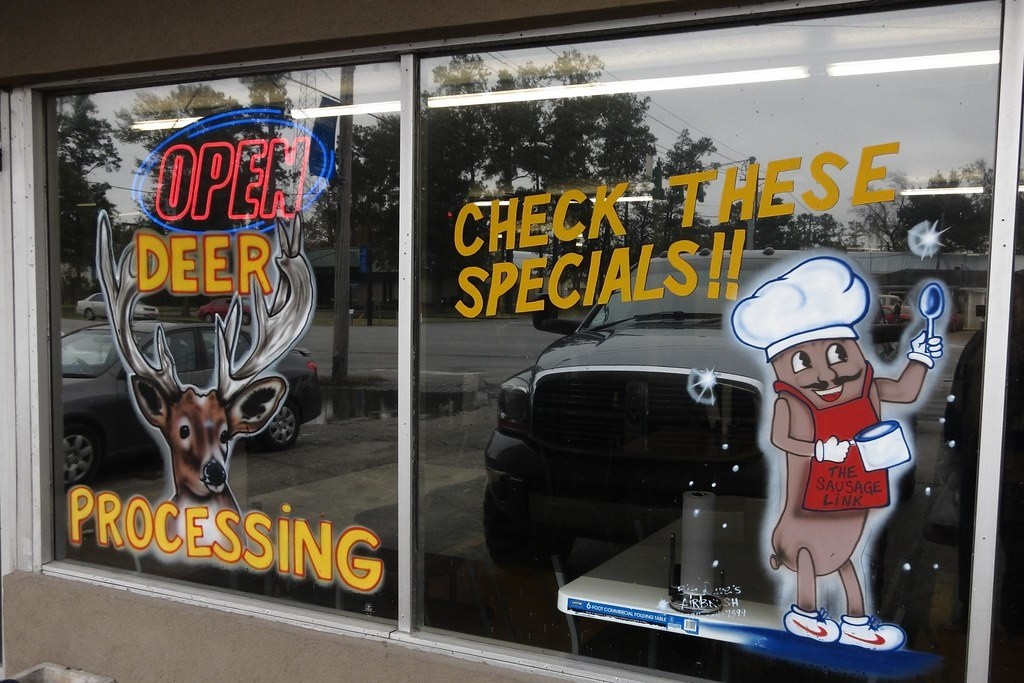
[199,297,252,326]
[60,322,322,494]
[883,305,916,325]
[77,292,160,322]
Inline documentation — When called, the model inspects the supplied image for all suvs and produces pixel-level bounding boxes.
[482,245,900,574]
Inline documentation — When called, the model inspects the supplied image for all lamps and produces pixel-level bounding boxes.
[826,50,1001,78]
[125,100,401,133]
[426,65,811,109]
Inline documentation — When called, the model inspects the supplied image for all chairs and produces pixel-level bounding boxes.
[550,519,714,680]
[758,558,912,683]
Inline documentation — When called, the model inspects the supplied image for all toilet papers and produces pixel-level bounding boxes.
[679,491,719,595]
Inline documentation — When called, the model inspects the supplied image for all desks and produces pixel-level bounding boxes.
[557,495,778,683]
